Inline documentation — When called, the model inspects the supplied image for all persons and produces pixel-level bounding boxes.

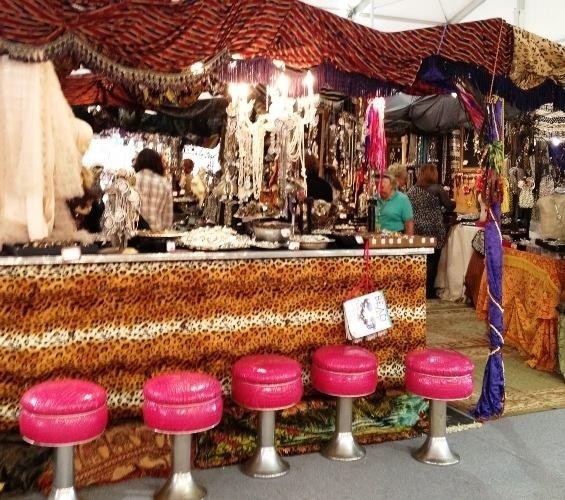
[179,159,205,207]
[299,155,456,299]
[133,149,173,254]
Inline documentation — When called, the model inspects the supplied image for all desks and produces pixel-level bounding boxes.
[477,246,564,376]
[434,220,484,302]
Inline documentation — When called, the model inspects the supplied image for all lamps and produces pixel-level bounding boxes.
[217,60,323,202]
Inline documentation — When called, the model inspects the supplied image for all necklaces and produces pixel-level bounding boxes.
[553,197,564,223]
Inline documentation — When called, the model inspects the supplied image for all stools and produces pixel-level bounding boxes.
[230,352,304,480]
[310,343,378,463]
[140,370,225,500]
[403,348,475,467]
[17,377,110,499]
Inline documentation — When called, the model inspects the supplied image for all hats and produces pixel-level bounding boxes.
[526,177,535,183]
[372,171,396,182]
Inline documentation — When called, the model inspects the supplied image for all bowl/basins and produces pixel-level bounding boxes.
[249,218,294,242]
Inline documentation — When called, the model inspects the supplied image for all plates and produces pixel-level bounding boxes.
[136,234,181,244]
[296,240,333,250]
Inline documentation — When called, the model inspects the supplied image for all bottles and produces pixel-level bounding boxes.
[367,198,376,233]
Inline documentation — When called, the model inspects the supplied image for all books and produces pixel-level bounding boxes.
[344,291,391,340]
